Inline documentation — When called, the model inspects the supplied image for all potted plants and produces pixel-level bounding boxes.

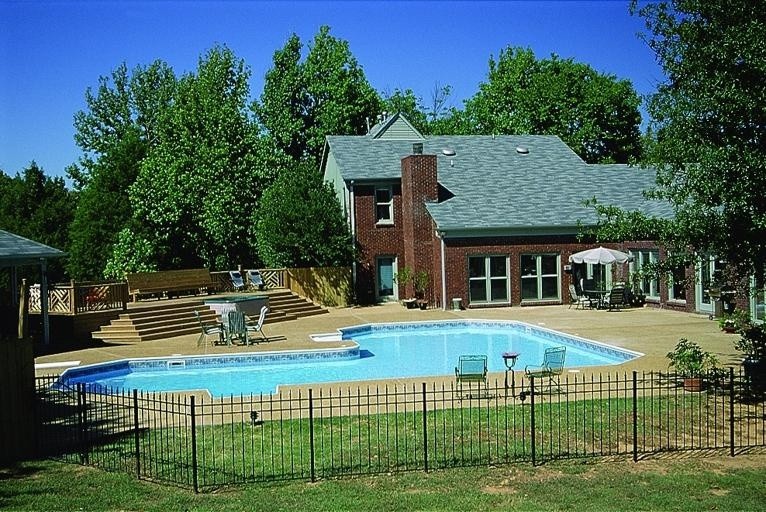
[664,338,721,393]
[393,266,431,311]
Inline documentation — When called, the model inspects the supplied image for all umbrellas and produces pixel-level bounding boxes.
[568,245,634,303]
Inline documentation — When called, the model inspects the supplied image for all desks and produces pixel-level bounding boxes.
[503,352,520,396]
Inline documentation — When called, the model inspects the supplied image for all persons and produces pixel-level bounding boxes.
[574,267,582,291]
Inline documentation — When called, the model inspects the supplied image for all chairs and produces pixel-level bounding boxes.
[453,355,488,399]
[567,278,624,312]
[228,270,267,292]
[192,303,269,348]
[520,345,567,402]
